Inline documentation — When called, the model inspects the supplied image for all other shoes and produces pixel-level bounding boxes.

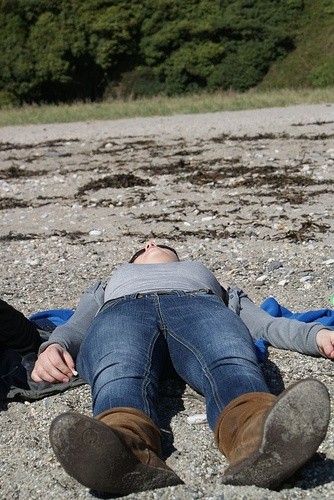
[216,379,329,487]
[50,407,180,495]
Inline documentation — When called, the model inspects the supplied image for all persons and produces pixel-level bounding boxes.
[32,242,334,495]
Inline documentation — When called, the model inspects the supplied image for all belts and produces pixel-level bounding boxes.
[121,291,207,298]
